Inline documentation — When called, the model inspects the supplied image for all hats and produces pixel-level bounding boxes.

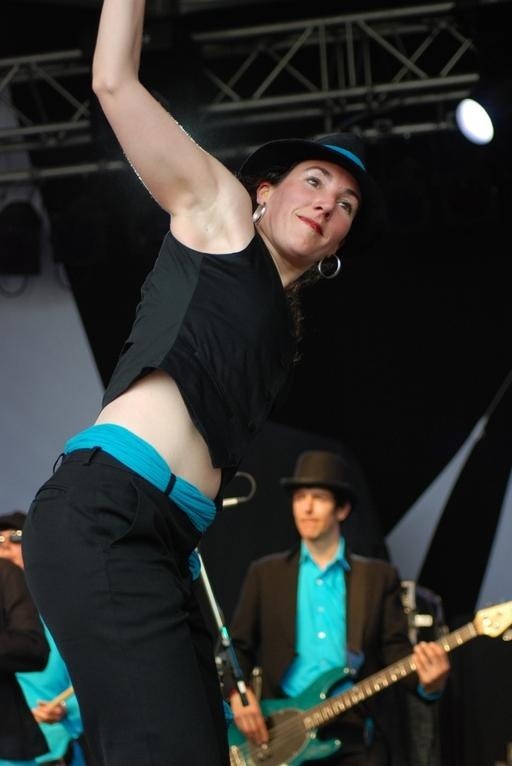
[279,449,359,498]
[237,131,386,254]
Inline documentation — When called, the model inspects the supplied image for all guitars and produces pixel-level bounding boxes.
[222,600,511,766]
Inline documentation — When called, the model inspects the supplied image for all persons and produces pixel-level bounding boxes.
[0,509,87,766]
[214,448,453,766]
[0,555,55,763]
[19,0,387,766]
